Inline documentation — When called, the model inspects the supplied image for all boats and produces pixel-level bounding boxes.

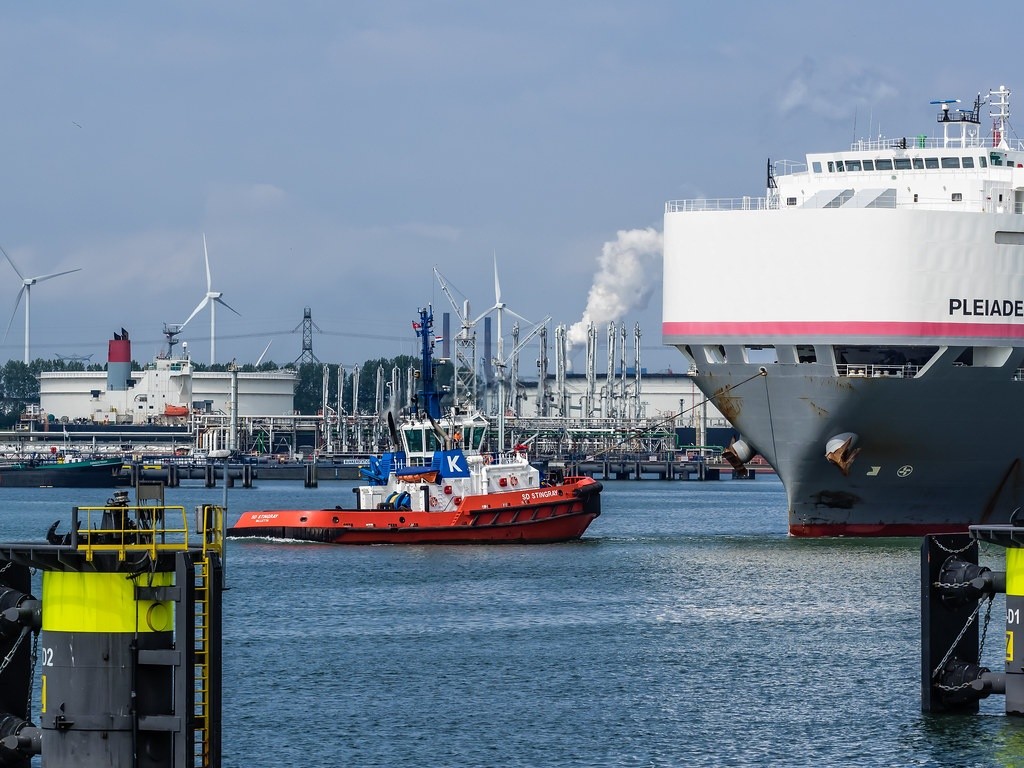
[0,451,124,488]
[663,83,1024,540]
[226,302,603,544]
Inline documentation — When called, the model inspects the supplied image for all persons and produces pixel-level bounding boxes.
[454,431,462,448]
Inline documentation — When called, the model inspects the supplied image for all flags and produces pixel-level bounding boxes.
[413,322,421,328]
[416,331,422,337]
[435,336,443,342]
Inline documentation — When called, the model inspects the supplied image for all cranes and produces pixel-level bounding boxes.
[431,267,553,418]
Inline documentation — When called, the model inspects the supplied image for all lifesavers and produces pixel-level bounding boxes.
[429,496,438,507]
[509,476,518,486]
